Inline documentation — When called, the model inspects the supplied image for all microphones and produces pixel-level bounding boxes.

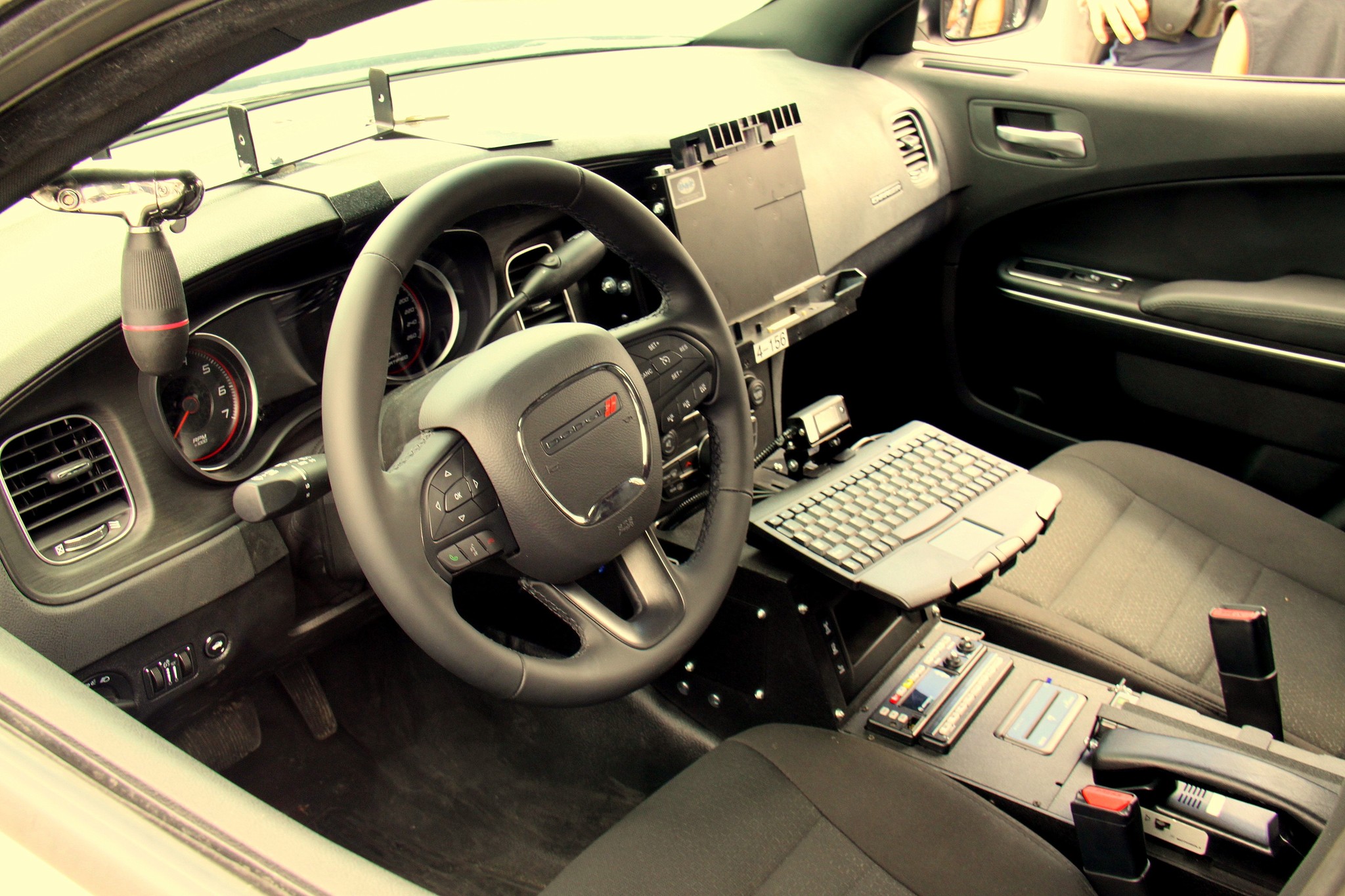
[117,219,192,370]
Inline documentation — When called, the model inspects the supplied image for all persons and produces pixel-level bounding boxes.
[946,0,1014,38]
[1082,0,1344,80]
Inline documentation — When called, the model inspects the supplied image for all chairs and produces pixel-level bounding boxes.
[537,723,1095,896]
[953,439,1345,757]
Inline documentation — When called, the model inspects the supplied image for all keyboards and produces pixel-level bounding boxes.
[740,419,1066,608]
